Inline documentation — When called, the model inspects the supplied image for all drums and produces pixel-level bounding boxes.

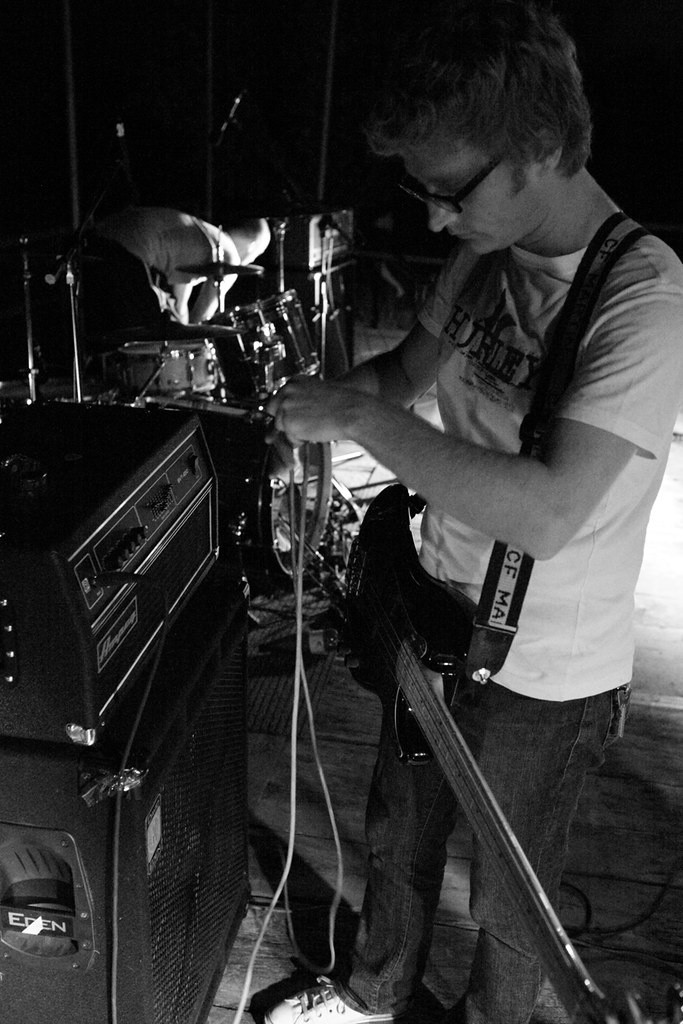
[117,335,220,395]
[207,288,320,403]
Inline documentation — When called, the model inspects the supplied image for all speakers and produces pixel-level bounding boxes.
[0,556,253,1024]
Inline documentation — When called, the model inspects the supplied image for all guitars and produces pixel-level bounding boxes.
[341,482,683,1024]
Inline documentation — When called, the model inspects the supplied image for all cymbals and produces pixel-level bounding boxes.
[175,261,265,277]
[107,318,250,341]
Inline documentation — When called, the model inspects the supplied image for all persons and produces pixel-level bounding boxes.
[261,0,683,1024]
[358,208,409,372]
[81,207,271,378]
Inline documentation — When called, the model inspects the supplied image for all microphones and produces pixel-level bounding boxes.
[214,90,245,147]
[116,122,130,171]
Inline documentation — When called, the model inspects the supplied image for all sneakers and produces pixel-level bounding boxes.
[263,974,410,1024]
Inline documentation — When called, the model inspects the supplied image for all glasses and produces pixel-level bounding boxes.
[400,135,520,216]
